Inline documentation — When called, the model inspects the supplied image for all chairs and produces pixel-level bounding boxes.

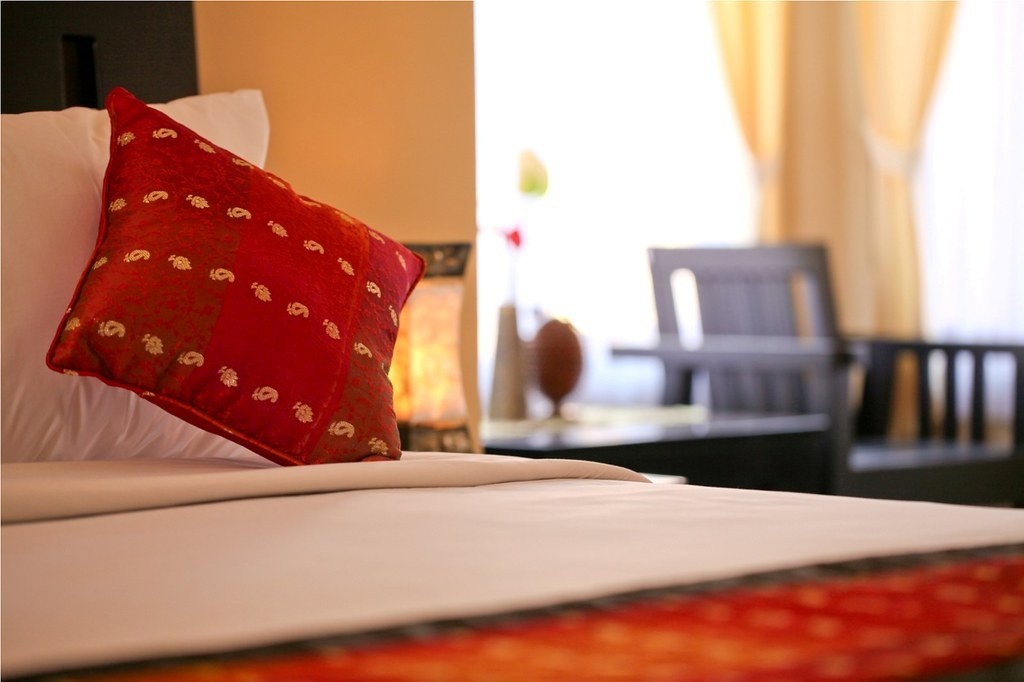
[607,239,1024,512]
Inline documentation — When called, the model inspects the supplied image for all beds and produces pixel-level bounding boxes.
[0,0,1024,682]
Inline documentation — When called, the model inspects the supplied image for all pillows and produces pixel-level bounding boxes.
[47,87,427,469]
[0,90,276,462]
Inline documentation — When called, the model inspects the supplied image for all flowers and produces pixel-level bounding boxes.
[500,148,550,300]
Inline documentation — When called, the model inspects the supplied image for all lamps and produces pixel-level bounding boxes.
[392,241,476,456]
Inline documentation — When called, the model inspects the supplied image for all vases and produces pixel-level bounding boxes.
[490,303,527,424]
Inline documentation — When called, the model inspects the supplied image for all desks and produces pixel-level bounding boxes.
[473,404,832,496]
